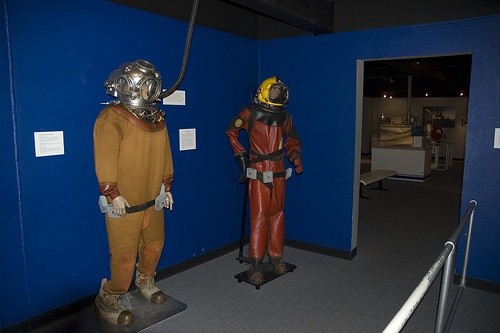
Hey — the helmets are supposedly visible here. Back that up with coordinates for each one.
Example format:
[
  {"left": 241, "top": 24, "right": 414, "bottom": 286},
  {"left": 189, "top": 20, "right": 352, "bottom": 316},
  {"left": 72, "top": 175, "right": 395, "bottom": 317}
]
[
  {"left": 103, "top": 59, "right": 162, "bottom": 120},
  {"left": 255, "top": 76, "right": 289, "bottom": 106}
]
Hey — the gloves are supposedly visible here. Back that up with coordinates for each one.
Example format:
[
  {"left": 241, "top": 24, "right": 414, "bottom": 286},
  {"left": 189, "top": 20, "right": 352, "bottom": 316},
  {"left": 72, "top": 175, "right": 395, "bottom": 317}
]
[
  {"left": 234, "top": 152, "right": 251, "bottom": 183},
  {"left": 290, "top": 152, "right": 303, "bottom": 174}
]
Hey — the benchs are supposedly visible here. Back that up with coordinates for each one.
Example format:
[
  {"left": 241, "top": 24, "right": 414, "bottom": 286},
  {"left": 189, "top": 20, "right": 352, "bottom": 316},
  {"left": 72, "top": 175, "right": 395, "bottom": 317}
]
[{"left": 359, "top": 169, "right": 397, "bottom": 200}]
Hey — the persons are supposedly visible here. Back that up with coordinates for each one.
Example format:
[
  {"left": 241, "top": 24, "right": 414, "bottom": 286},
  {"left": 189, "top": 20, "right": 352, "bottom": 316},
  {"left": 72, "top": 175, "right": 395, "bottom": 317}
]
[
  {"left": 93, "top": 60, "right": 173, "bottom": 326},
  {"left": 225, "top": 75, "right": 303, "bottom": 284}
]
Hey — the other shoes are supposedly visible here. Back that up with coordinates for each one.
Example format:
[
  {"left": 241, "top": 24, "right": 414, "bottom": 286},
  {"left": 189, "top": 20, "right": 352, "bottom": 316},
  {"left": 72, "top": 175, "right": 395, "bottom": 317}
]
[
  {"left": 248, "top": 258, "right": 264, "bottom": 285},
  {"left": 95, "top": 278, "right": 135, "bottom": 326},
  {"left": 269, "top": 256, "right": 286, "bottom": 273},
  {"left": 134, "top": 271, "right": 167, "bottom": 305}
]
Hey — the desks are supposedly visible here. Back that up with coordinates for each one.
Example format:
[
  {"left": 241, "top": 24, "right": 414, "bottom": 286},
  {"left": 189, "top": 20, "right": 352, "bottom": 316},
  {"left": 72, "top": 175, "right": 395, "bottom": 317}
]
[{"left": 371, "top": 147, "right": 432, "bottom": 182}]
